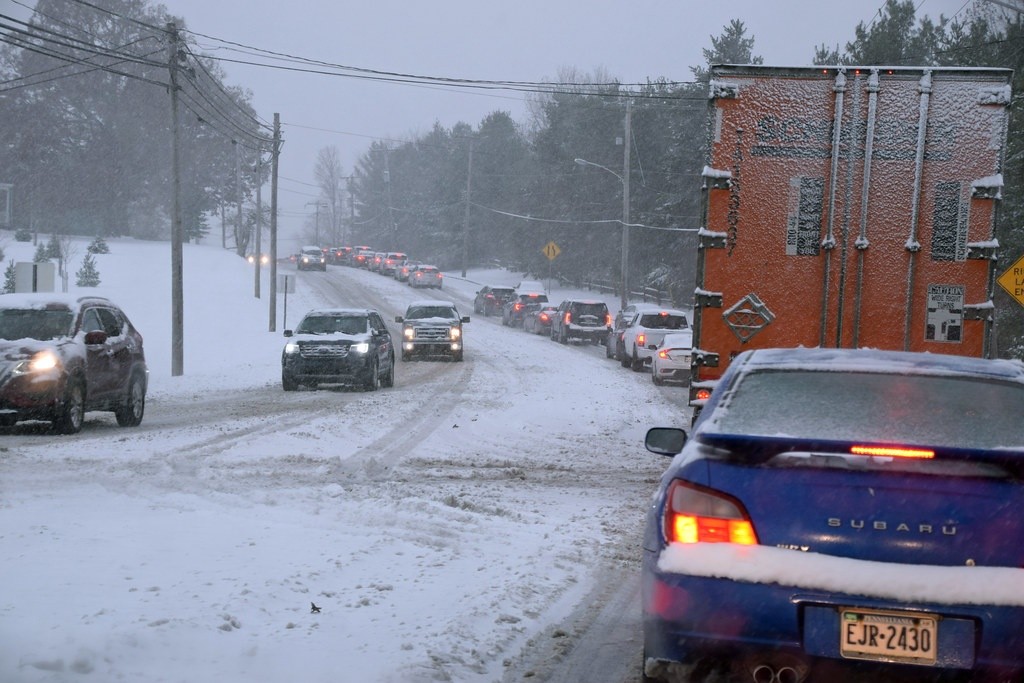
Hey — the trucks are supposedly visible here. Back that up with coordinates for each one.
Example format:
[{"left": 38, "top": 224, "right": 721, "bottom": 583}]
[{"left": 688, "top": 63, "right": 1015, "bottom": 431}]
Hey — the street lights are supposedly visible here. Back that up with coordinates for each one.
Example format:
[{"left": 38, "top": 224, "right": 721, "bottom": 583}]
[{"left": 574, "top": 97, "right": 632, "bottom": 310}]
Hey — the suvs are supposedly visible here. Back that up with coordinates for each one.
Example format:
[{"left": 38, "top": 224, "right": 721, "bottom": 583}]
[
  {"left": 502, "top": 291, "right": 549, "bottom": 328},
  {"left": 473, "top": 285, "right": 516, "bottom": 317},
  {"left": 281, "top": 309, "right": 395, "bottom": 392},
  {"left": 297, "top": 246, "right": 326, "bottom": 270},
  {"left": 550, "top": 300, "right": 612, "bottom": 346},
  {"left": 1, "top": 293, "right": 148, "bottom": 435},
  {"left": 620, "top": 310, "right": 693, "bottom": 372},
  {"left": 396, "top": 299, "right": 472, "bottom": 363}
]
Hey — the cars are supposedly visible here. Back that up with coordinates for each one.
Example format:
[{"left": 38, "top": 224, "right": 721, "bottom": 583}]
[
  {"left": 605, "top": 316, "right": 635, "bottom": 361},
  {"left": 642, "top": 348, "right": 1024, "bottom": 683},
  {"left": 614, "top": 302, "right": 662, "bottom": 326},
  {"left": 524, "top": 303, "right": 560, "bottom": 335},
  {"left": 648, "top": 334, "right": 692, "bottom": 388},
  {"left": 322, "top": 246, "right": 443, "bottom": 289}
]
